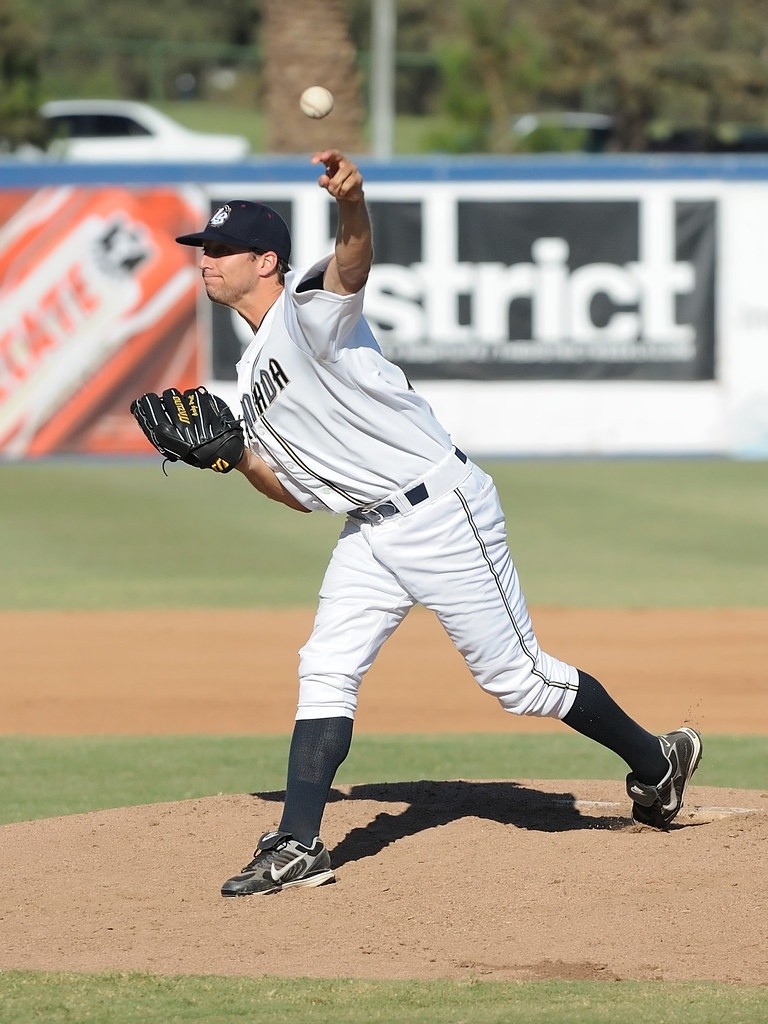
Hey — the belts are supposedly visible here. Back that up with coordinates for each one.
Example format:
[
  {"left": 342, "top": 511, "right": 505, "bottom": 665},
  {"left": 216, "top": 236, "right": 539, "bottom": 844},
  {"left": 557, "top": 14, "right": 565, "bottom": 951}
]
[{"left": 347, "top": 445, "right": 468, "bottom": 524}]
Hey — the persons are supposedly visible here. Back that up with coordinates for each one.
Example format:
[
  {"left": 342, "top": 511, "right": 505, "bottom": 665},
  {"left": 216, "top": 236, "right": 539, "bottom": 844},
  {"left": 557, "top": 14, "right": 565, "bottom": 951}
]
[{"left": 131, "top": 148, "right": 702, "bottom": 897}]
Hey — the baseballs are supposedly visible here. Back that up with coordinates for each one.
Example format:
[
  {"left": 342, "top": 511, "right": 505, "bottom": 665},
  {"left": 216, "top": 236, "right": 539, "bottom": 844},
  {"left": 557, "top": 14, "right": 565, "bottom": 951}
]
[{"left": 301, "top": 84, "right": 334, "bottom": 119}]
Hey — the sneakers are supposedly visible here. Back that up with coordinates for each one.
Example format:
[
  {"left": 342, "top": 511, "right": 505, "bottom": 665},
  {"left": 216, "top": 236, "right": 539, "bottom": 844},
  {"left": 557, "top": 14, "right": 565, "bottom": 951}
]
[
  {"left": 626, "top": 725, "right": 703, "bottom": 829},
  {"left": 222, "top": 830, "right": 335, "bottom": 900}
]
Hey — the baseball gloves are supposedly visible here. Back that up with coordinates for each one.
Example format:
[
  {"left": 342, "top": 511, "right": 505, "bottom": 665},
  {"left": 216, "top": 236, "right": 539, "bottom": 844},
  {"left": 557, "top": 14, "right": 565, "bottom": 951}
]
[{"left": 130, "top": 387, "right": 245, "bottom": 475}]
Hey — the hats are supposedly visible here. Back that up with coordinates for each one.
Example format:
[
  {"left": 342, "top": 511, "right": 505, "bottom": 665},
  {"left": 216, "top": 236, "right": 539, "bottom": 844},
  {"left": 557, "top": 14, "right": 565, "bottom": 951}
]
[{"left": 174, "top": 200, "right": 292, "bottom": 263}]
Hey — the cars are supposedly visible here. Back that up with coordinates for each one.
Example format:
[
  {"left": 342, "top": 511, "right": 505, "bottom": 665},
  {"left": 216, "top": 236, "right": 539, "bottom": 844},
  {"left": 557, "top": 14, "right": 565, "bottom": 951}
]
[
  {"left": 2, "top": 96, "right": 255, "bottom": 173},
  {"left": 460, "top": 112, "right": 767, "bottom": 156}
]
